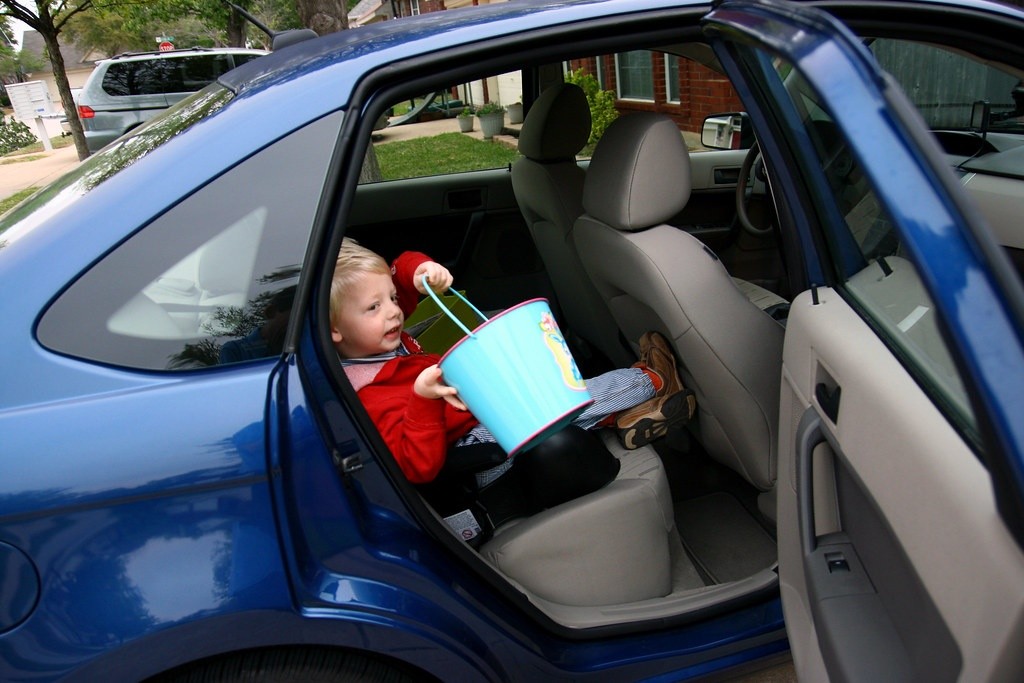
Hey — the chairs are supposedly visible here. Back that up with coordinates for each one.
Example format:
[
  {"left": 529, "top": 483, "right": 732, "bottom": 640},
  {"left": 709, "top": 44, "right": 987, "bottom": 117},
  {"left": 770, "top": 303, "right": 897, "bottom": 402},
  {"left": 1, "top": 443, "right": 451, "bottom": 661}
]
[{"left": 194, "top": 83, "right": 793, "bottom": 608}]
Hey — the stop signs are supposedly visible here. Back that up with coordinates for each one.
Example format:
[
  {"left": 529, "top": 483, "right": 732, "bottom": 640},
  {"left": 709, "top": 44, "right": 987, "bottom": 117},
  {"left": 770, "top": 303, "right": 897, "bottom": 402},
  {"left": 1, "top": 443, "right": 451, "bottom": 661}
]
[{"left": 159, "top": 41, "right": 174, "bottom": 51}]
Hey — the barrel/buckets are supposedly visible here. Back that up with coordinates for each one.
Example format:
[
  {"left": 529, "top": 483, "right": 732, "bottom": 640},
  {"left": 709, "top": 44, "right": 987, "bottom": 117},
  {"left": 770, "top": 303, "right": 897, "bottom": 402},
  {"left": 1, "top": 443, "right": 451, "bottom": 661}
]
[{"left": 421, "top": 274, "right": 596, "bottom": 459}]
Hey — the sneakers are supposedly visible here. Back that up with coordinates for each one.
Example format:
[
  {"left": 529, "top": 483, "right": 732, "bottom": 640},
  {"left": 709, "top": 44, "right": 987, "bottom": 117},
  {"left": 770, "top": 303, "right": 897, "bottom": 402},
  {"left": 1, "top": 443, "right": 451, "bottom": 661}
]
[
  {"left": 613, "top": 389, "right": 696, "bottom": 450},
  {"left": 629, "top": 330, "right": 684, "bottom": 398}
]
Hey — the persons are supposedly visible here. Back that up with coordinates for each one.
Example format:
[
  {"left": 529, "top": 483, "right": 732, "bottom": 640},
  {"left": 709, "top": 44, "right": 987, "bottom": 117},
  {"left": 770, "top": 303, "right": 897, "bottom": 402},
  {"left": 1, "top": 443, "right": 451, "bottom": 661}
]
[{"left": 331, "top": 240, "right": 697, "bottom": 487}]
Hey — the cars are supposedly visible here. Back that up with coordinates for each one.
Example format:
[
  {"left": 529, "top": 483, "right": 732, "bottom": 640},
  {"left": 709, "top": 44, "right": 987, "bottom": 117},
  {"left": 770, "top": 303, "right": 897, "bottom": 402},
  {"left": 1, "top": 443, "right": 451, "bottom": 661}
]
[{"left": 0, "top": 0, "right": 1024, "bottom": 683}]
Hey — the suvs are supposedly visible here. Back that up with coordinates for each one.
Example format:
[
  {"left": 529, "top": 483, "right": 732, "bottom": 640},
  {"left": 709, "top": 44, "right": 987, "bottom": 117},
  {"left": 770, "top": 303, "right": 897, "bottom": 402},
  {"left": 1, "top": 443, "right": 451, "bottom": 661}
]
[{"left": 77, "top": 44, "right": 274, "bottom": 155}]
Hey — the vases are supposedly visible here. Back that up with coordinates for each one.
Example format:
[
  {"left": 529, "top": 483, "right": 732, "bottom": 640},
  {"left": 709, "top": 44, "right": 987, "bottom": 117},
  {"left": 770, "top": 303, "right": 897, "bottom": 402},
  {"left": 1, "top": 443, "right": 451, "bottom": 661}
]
[{"left": 505, "top": 103, "right": 524, "bottom": 124}]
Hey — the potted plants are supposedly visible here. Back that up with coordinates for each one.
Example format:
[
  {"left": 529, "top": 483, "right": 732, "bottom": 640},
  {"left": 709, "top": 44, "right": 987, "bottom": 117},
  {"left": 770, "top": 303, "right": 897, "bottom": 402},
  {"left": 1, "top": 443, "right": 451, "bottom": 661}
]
[
  {"left": 455, "top": 106, "right": 475, "bottom": 133},
  {"left": 476, "top": 100, "right": 507, "bottom": 139}
]
